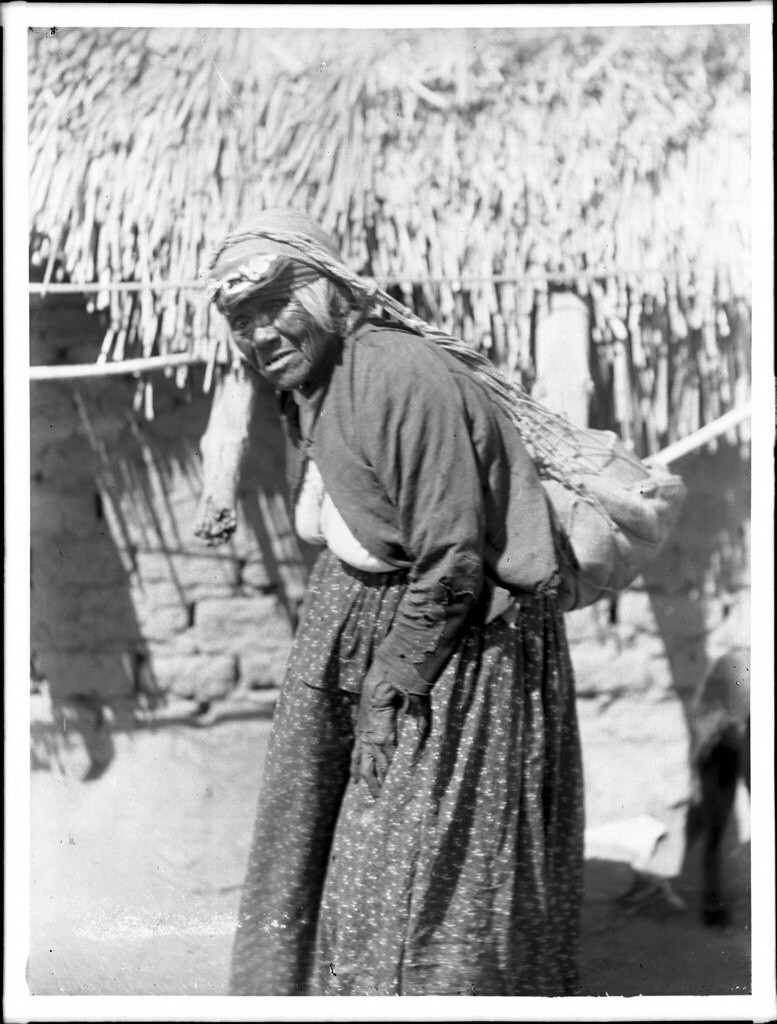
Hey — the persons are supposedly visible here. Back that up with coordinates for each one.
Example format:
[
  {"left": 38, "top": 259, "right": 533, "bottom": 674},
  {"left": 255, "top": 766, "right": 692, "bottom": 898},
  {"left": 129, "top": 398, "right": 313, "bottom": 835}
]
[{"left": 204, "top": 204, "right": 586, "bottom": 996}]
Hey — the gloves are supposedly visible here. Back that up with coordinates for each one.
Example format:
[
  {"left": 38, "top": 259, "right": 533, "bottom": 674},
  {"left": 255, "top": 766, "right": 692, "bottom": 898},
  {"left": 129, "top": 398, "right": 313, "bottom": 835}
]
[{"left": 345, "top": 657, "right": 399, "bottom": 797}]
